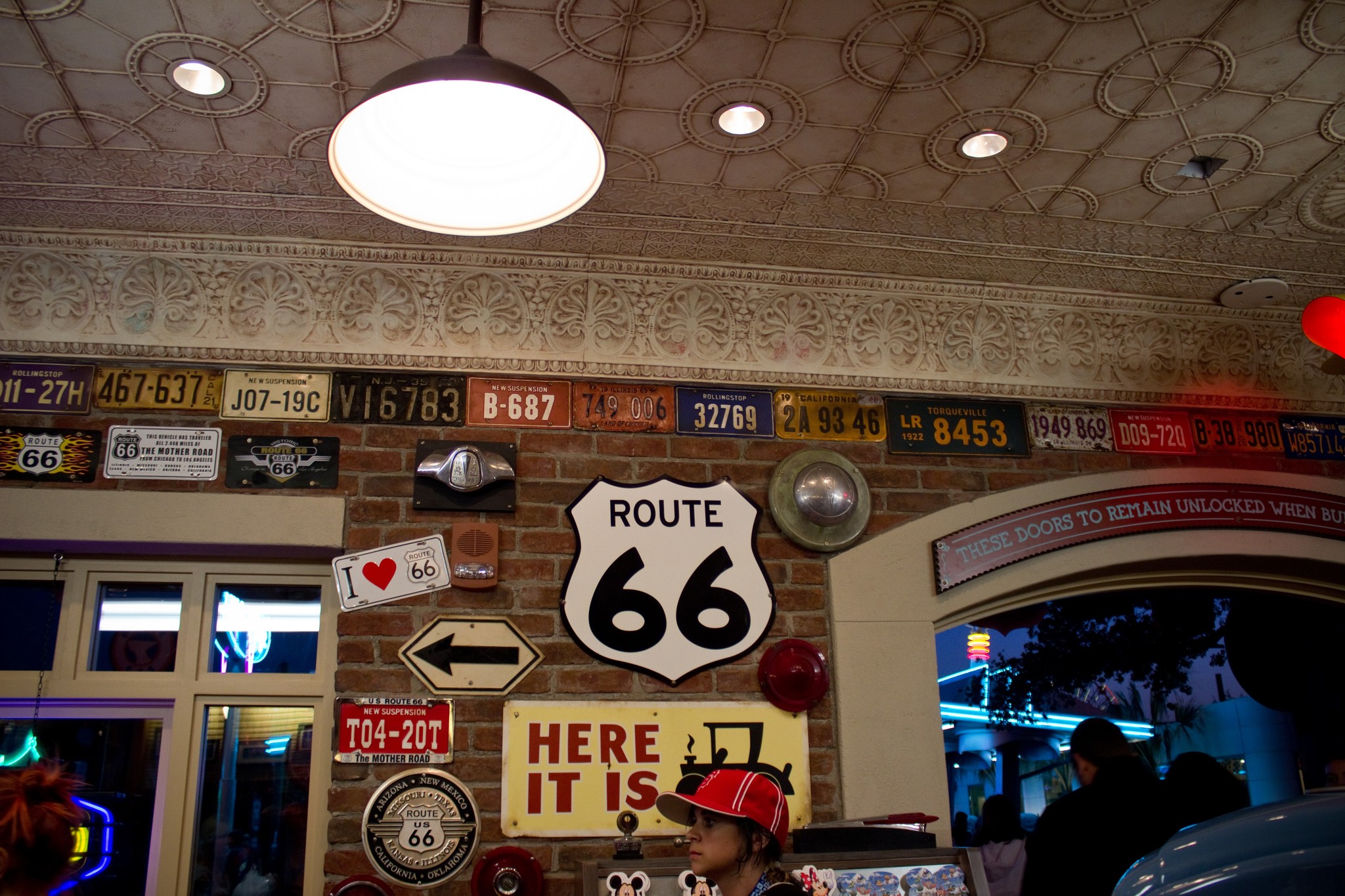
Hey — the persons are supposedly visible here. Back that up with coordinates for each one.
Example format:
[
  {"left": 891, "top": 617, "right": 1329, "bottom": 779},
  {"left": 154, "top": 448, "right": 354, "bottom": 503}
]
[
  {"left": 655, "top": 770, "right": 809, "bottom": 895},
  {"left": 0, "top": 757, "right": 85, "bottom": 896},
  {"left": 954, "top": 713, "right": 1247, "bottom": 895}
]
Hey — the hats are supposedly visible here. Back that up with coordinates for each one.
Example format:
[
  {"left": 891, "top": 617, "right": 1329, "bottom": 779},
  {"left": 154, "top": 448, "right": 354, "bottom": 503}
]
[{"left": 657, "top": 769, "right": 787, "bottom": 850}]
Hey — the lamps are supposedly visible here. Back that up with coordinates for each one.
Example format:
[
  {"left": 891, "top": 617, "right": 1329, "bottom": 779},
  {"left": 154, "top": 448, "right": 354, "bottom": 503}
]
[{"left": 327, "top": 1, "right": 607, "bottom": 237}]
